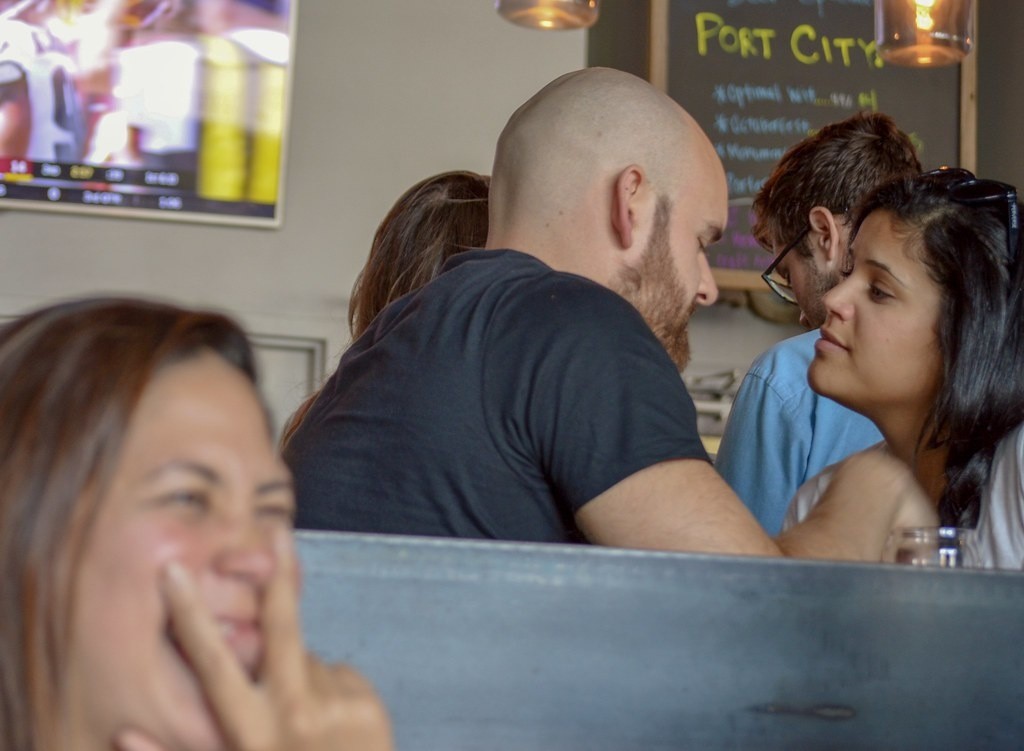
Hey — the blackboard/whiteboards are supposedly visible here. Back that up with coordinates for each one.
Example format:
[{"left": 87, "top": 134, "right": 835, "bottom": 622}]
[{"left": 650, "top": 0, "right": 978, "bottom": 290}]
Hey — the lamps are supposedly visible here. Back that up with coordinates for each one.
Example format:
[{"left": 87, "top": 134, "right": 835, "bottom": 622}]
[
  {"left": 492, "top": 0, "right": 599, "bottom": 30},
  {"left": 871, "top": 0, "right": 973, "bottom": 67}
]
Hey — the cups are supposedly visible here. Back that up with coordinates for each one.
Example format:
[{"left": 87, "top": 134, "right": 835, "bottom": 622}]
[{"left": 877, "top": 527, "right": 987, "bottom": 567}]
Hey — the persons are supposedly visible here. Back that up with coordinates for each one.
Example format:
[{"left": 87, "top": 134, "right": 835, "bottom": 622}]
[
  {"left": 0, "top": 296, "right": 393, "bottom": 751},
  {"left": 715, "top": 113, "right": 922, "bottom": 538},
  {"left": 782, "top": 168, "right": 1024, "bottom": 569},
  {"left": 279, "top": 66, "right": 941, "bottom": 563},
  {"left": 280, "top": 169, "right": 489, "bottom": 444}
]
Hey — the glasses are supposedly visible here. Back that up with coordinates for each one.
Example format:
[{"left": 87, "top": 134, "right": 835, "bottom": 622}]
[
  {"left": 759, "top": 206, "right": 849, "bottom": 305},
  {"left": 924, "top": 165, "right": 1019, "bottom": 282}
]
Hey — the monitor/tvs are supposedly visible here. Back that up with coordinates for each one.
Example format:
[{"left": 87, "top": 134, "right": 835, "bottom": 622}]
[{"left": 0, "top": 0, "right": 302, "bottom": 228}]
